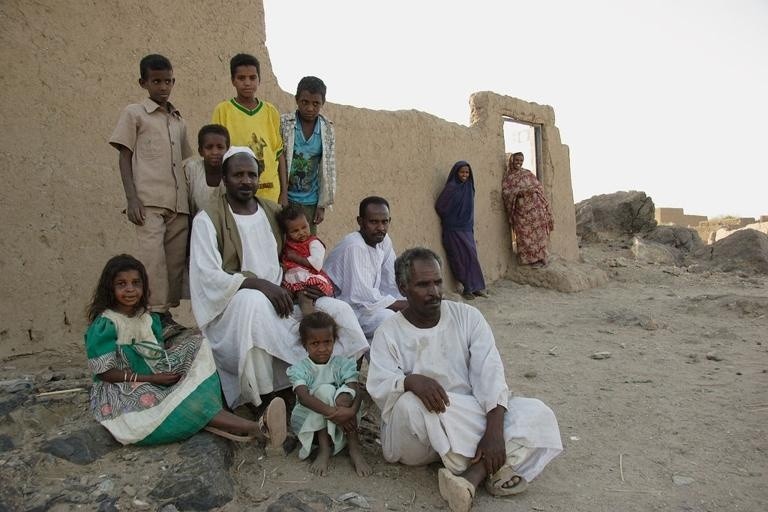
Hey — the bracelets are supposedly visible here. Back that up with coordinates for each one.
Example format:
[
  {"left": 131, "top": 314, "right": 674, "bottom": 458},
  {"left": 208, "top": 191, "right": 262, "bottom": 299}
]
[
  {"left": 134, "top": 372, "right": 138, "bottom": 383},
  {"left": 130, "top": 372, "right": 135, "bottom": 382},
  {"left": 123, "top": 370, "right": 128, "bottom": 383}
]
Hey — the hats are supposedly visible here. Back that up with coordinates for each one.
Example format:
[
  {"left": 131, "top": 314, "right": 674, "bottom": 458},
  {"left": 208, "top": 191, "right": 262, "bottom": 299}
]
[{"left": 222, "top": 145, "right": 257, "bottom": 164}]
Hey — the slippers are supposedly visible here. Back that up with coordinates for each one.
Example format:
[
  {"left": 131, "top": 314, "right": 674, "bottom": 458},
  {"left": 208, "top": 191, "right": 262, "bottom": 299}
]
[
  {"left": 485, "top": 465, "right": 528, "bottom": 496},
  {"left": 259, "top": 397, "right": 287, "bottom": 449},
  {"left": 438, "top": 468, "right": 475, "bottom": 511},
  {"left": 204, "top": 426, "right": 254, "bottom": 443}
]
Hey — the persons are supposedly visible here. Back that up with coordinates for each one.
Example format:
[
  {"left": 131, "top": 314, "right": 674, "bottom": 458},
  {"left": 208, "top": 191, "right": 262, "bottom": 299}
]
[
  {"left": 189, "top": 141, "right": 373, "bottom": 408},
  {"left": 323, "top": 195, "right": 412, "bottom": 351},
  {"left": 279, "top": 75, "right": 337, "bottom": 236},
  {"left": 272, "top": 203, "right": 337, "bottom": 316},
  {"left": 209, "top": 53, "right": 291, "bottom": 211},
  {"left": 82, "top": 252, "right": 287, "bottom": 449},
  {"left": 435, "top": 159, "right": 490, "bottom": 300},
  {"left": 181, "top": 124, "right": 231, "bottom": 219},
  {"left": 285, "top": 310, "right": 373, "bottom": 479},
  {"left": 501, "top": 152, "right": 554, "bottom": 269},
  {"left": 364, "top": 244, "right": 566, "bottom": 512},
  {"left": 106, "top": 53, "right": 199, "bottom": 339}
]
